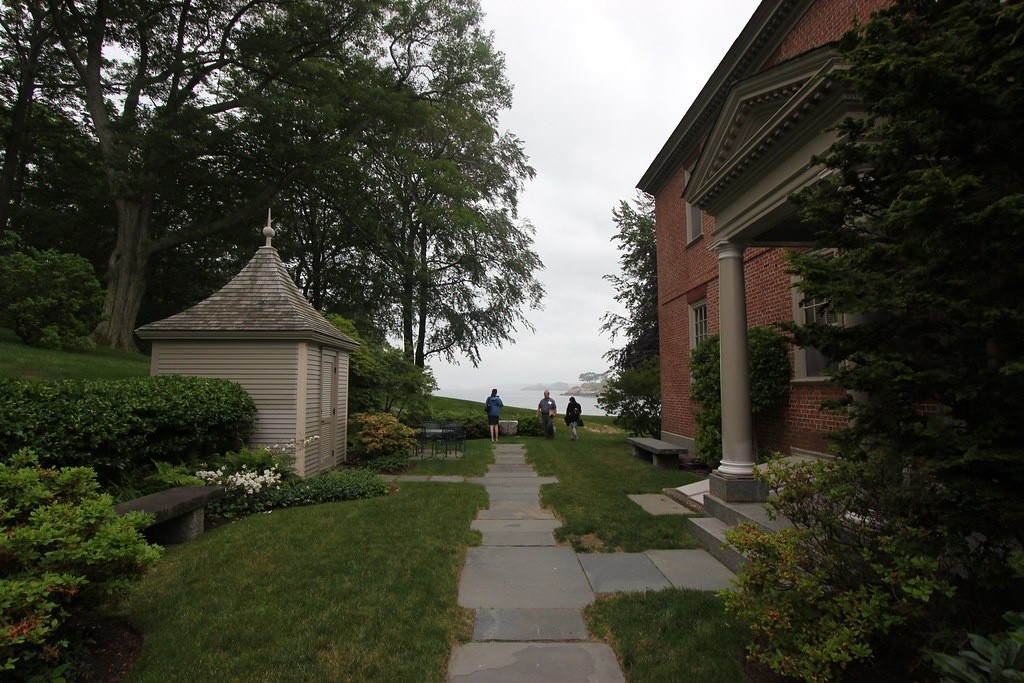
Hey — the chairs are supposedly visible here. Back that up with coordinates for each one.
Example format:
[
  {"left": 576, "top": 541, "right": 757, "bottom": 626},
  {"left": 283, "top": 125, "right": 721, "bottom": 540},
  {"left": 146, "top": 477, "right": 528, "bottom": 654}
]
[
  {"left": 407, "top": 425, "right": 427, "bottom": 461},
  {"left": 433, "top": 425, "right": 457, "bottom": 461}
]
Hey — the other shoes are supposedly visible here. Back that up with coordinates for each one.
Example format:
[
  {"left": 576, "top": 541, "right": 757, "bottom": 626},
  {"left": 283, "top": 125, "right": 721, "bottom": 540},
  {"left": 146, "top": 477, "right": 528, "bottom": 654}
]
[
  {"left": 548, "top": 435, "right": 554, "bottom": 439},
  {"left": 545, "top": 435, "right": 549, "bottom": 438}
]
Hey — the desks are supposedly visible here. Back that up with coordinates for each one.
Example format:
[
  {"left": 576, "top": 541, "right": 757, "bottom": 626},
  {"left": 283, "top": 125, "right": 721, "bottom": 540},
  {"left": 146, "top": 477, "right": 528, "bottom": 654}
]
[{"left": 417, "top": 429, "right": 455, "bottom": 461}]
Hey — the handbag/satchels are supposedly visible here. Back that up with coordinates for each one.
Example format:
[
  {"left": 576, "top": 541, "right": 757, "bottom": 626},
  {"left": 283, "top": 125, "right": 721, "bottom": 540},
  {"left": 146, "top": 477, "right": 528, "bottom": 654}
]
[
  {"left": 484, "top": 404, "right": 492, "bottom": 412},
  {"left": 577, "top": 419, "right": 584, "bottom": 427}
]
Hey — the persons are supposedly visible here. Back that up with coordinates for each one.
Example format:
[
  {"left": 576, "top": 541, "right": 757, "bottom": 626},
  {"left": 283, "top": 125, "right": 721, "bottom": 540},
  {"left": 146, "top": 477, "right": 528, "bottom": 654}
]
[
  {"left": 486, "top": 389, "right": 503, "bottom": 442},
  {"left": 536, "top": 390, "right": 557, "bottom": 439},
  {"left": 566, "top": 397, "right": 581, "bottom": 440}
]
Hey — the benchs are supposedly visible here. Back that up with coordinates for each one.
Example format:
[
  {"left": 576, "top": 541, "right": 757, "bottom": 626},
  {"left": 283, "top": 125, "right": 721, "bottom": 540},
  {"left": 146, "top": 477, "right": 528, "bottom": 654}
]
[
  {"left": 627, "top": 438, "right": 688, "bottom": 467},
  {"left": 113, "top": 486, "right": 226, "bottom": 542}
]
